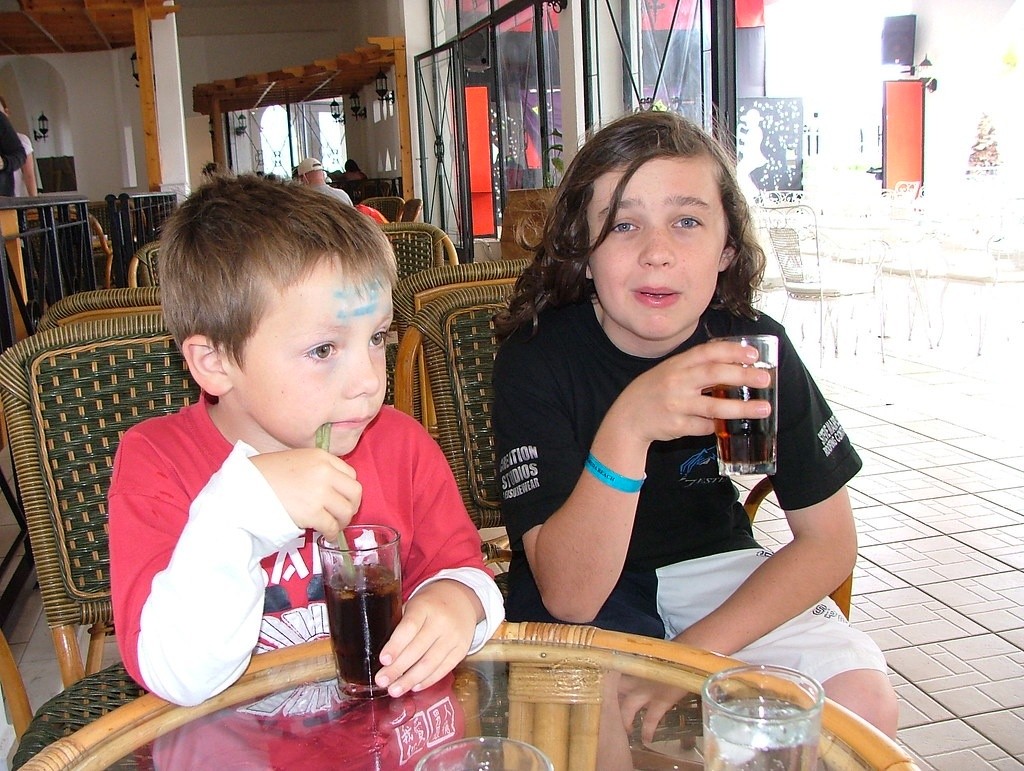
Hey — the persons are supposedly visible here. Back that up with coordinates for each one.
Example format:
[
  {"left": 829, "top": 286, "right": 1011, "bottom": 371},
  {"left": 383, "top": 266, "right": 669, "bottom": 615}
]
[
  {"left": 108, "top": 174, "right": 504, "bottom": 771},
  {"left": 491, "top": 109, "right": 898, "bottom": 771},
  {"left": 0, "top": 95, "right": 38, "bottom": 232},
  {"left": 257, "top": 159, "right": 368, "bottom": 208}
]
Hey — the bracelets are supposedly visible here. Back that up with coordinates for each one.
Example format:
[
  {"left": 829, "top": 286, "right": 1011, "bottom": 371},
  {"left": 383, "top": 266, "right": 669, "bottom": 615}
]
[{"left": 586, "top": 452, "right": 647, "bottom": 493}]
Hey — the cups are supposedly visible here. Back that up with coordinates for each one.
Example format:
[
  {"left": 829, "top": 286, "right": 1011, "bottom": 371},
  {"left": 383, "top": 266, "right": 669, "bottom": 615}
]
[
  {"left": 701, "top": 664, "right": 826, "bottom": 771},
  {"left": 707, "top": 333, "right": 779, "bottom": 475},
  {"left": 316, "top": 525, "right": 403, "bottom": 700}
]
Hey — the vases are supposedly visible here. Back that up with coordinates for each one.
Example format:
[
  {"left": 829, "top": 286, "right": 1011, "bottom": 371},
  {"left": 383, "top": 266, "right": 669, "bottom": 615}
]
[{"left": 544, "top": 172, "right": 554, "bottom": 189}]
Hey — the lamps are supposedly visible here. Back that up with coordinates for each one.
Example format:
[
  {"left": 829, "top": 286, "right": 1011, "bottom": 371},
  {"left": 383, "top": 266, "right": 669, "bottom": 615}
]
[
  {"left": 919, "top": 54, "right": 937, "bottom": 93},
  {"left": 375, "top": 66, "right": 395, "bottom": 106},
  {"left": 131, "top": 51, "right": 139, "bottom": 88},
  {"left": 34, "top": 111, "right": 49, "bottom": 142},
  {"left": 349, "top": 88, "right": 366, "bottom": 120},
  {"left": 330, "top": 100, "right": 346, "bottom": 125},
  {"left": 236, "top": 113, "right": 248, "bottom": 136}
]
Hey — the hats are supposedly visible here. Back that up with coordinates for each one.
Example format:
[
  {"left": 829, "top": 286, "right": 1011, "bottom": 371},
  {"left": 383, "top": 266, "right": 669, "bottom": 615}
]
[{"left": 298, "top": 157, "right": 323, "bottom": 176}]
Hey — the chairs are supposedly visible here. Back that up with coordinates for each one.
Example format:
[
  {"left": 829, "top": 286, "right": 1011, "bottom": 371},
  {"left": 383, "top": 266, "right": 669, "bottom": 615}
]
[{"left": 0, "top": 177, "right": 1024, "bottom": 771}]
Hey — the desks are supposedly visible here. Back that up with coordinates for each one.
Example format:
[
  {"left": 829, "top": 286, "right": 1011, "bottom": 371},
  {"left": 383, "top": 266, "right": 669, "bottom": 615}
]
[{"left": 16, "top": 622, "right": 919, "bottom": 771}]
